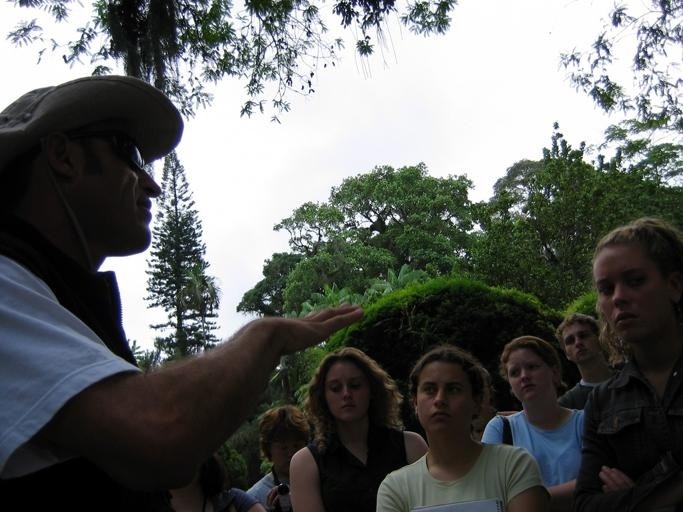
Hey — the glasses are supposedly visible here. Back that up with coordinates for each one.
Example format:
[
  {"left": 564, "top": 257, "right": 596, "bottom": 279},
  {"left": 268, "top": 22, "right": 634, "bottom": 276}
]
[{"left": 68, "top": 129, "right": 146, "bottom": 171}]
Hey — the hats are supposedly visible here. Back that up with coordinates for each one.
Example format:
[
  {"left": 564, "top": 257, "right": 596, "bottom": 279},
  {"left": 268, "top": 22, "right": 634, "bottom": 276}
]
[{"left": 0, "top": 75, "right": 183, "bottom": 172}]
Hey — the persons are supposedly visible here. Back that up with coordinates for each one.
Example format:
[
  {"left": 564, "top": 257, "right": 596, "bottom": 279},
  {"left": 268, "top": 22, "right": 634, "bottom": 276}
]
[
  {"left": 172, "top": 217, "right": 682, "bottom": 511},
  {"left": 0, "top": 76, "right": 364, "bottom": 512}
]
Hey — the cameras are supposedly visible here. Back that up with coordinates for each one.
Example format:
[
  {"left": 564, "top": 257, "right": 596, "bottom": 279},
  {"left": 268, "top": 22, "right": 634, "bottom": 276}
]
[{"left": 274, "top": 484, "right": 291, "bottom": 511}]
